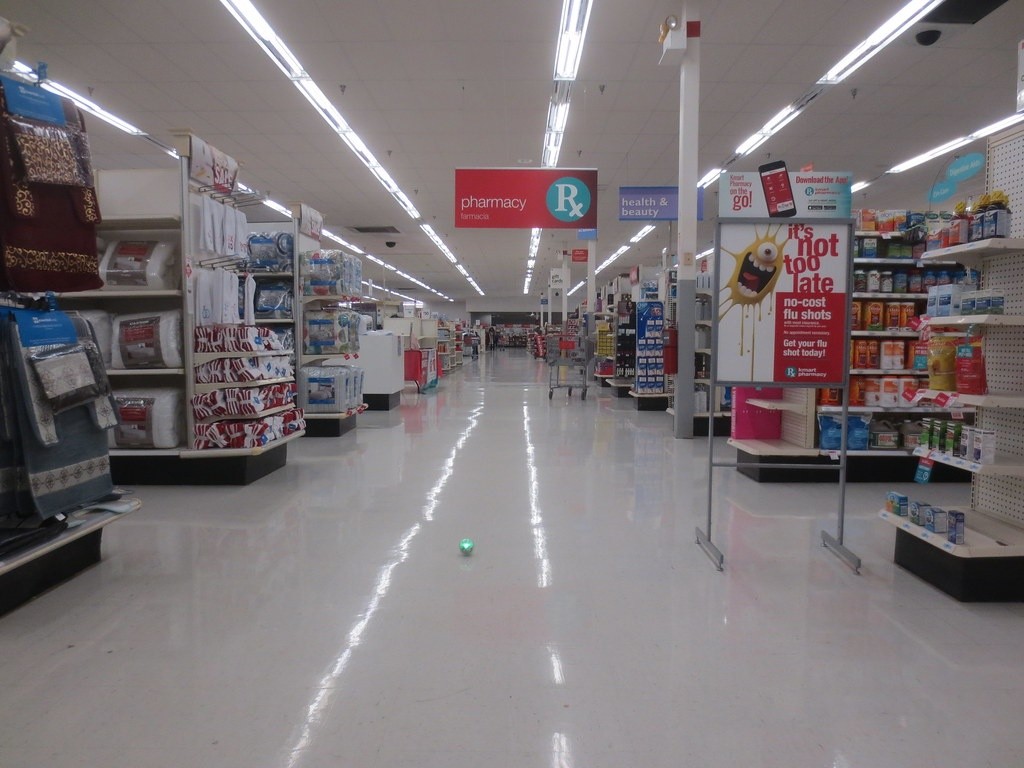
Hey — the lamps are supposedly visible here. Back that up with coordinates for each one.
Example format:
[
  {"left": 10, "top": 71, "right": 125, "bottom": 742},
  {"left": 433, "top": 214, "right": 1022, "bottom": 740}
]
[
  {"left": 289, "top": 77, "right": 352, "bottom": 133},
  {"left": 420, "top": 224, "right": 457, "bottom": 264},
  {"left": 10, "top": 61, "right": 293, "bottom": 218},
  {"left": 697, "top": 169, "right": 728, "bottom": 189},
  {"left": 735, "top": 105, "right": 801, "bottom": 156},
  {"left": 367, "top": 166, "right": 401, "bottom": 193},
  {"left": 595, "top": 246, "right": 630, "bottom": 276},
  {"left": 815, "top": 0, "right": 947, "bottom": 84},
  {"left": 391, "top": 190, "right": 421, "bottom": 220},
  {"left": 658, "top": 15, "right": 677, "bottom": 43},
  {"left": 529, "top": 227, "right": 542, "bottom": 258},
  {"left": 219, "top": 0, "right": 311, "bottom": 81},
  {"left": 336, "top": 126, "right": 383, "bottom": 168},
  {"left": 630, "top": 225, "right": 656, "bottom": 243}
]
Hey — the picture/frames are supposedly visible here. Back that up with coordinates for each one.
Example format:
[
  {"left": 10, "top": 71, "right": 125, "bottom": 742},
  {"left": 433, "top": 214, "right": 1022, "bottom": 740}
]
[{"left": 710, "top": 216, "right": 855, "bottom": 389}]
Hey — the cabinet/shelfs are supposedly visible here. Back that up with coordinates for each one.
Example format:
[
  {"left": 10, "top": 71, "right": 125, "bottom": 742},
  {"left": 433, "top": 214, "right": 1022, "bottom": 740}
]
[
  {"left": 237, "top": 221, "right": 297, "bottom": 410},
  {"left": 328, "top": 252, "right": 733, "bottom": 438},
  {"left": 0, "top": 167, "right": 186, "bottom": 455},
  {"left": 296, "top": 231, "right": 369, "bottom": 419},
  {"left": 877, "top": 120, "right": 1023, "bottom": 560},
  {"left": 180, "top": 155, "right": 305, "bottom": 458},
  {"left": 727, "top": 387, "right": 816, "bottom": 455},
  {"left": 813, "top": 232, "right": 967, "bottom": 456}
]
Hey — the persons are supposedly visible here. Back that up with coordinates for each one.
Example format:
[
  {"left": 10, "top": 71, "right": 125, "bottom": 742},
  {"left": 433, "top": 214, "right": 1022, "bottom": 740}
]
[{"left": 489, "top": 327, "right": 496, "bottom": 350}]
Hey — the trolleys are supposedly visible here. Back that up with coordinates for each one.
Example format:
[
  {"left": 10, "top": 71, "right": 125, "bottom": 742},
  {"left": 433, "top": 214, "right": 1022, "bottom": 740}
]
[
  {"left": 498, "top": 334, "right": 507, "bottom": 352},
  {"left": 546, "top": 332, "right": 590, "bottom": 401}
]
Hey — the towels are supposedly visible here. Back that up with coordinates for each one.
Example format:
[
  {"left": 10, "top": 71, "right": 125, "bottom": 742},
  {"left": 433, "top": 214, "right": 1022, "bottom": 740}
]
[
  {"left": 244, "top": 273, "right": 256, "bottom": 325},
  {"left": 196, "top": 267, "right": 240, "bottom": 325},
  {"left": 197, "top": 196, "right": 249, "bottom": 259}
]
